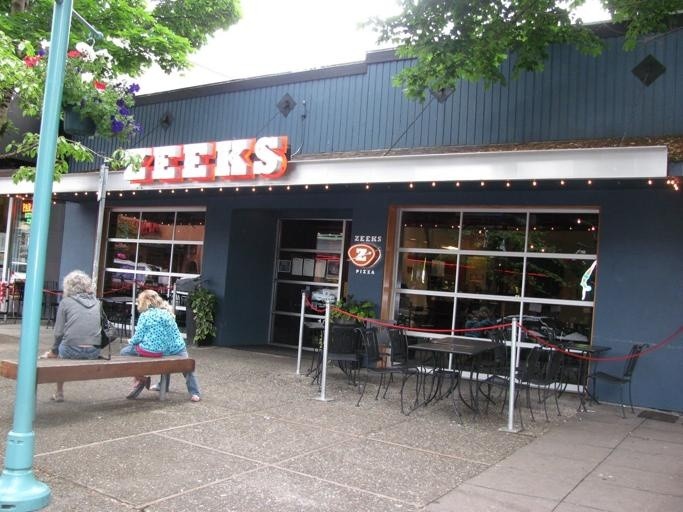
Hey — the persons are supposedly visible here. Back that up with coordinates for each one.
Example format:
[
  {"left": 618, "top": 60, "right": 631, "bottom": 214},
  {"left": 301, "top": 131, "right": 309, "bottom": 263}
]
[
  {"left": 117, "top": 287, "right": 179, "bottom": 402},
  {"left": 36, "top": 268, "right": 103, "bottom": 405},
  {"left": 465, "top": 307, "right": 496, "bottom": 339},
  {"left": 146, "top": 300, "right": 201, "bottom": 401}
]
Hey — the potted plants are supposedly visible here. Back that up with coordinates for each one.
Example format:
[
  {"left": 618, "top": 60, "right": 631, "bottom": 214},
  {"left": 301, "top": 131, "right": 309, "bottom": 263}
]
[{"left": 190, "top": 283, "right": 218, "bottom": 348}]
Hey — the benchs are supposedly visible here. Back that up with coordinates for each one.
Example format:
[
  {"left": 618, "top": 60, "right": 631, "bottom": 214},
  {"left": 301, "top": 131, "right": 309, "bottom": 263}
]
[{"left": 0, "top": 351, "right": 198, "bottom": 403}]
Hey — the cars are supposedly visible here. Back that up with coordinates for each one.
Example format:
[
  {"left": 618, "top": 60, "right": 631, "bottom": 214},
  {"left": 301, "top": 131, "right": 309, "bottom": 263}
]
[
  {"left": 110, "top": 259, "right": 201, "bottom": 325},
  {"left": 496, "top": 314, "right": 589, "bottom": 360},
  {"left": 11, "top": 261, "right": 28, "bottom": 282},
  {"left": 309, "top": 290, "right": 339, "bottom": 309},
  {"left": 395, "top": 294, "right": 423, "bottom": 364}
]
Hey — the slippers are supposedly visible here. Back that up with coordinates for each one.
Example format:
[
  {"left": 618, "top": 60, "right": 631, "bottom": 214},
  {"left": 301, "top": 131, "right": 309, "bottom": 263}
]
[
  {"left": 51, "top": 392, "right": 65, "bottom": 402},
  {"left": 38, "top": 350, "right": 63, "bottom": 359}
]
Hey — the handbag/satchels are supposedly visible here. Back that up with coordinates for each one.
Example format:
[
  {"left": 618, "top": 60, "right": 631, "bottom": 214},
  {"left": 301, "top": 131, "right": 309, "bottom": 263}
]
[{"left": 100, "top": 304, "right": 118, "bottom": 348}]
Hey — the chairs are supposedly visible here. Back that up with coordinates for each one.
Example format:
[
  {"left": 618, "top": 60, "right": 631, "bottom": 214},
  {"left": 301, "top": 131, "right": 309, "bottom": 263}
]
[{"left": 13, "top": 279, "right": 170, "bottom": 344}]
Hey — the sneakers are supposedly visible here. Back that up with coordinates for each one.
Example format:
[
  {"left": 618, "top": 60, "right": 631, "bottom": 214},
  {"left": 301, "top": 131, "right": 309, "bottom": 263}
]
[
  {"left": 145, "top": 376, "right": 152, "bottom": 392},
  {"left": 191, "top": 395, "right": 200, "bottom": 402},
  {"left": 150, "top": 384, "right": 168, "bottom": 391},
  {"left": 127, "top": 385, "right": 145, "bottom": 400}
]
